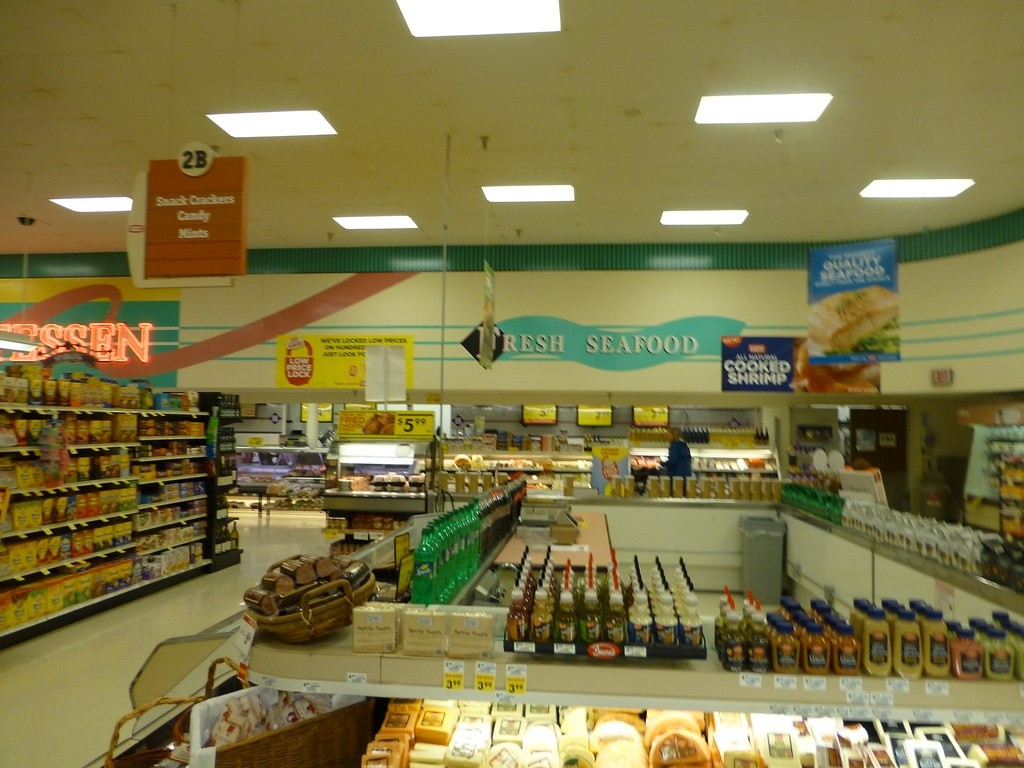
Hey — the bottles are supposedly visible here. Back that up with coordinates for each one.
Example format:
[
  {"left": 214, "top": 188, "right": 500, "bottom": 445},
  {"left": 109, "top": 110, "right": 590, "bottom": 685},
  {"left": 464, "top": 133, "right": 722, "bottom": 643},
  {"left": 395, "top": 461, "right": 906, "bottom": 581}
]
[
  {"left": 627, "top": 425, "right": 769, "bottom": 445},
  {"left": 219, "top": 427, "right": 236, "bottom": 453},
  {"left": 714, "top": 586, "right": 1024, "bottom": 680},
  {"left": 412, "top": 476, "right": 703, "bottom": 647},
  {"left": 217, "top": 458, "right": 237, "bottom": 488},
  {"left": 220, "top": 394, "right": 241, "bottom": 416},
  {"left": 584, "top": 433, "right": 602, "bottom": 451},
  {"left": 215, "top": 522, "right": 239, "bottom": 555},
  {"left": 217, "top": 496, "right": 228, "bottom": 521},
  {"left": 553, "top": 429, "right": 568, "bottom": 453}
]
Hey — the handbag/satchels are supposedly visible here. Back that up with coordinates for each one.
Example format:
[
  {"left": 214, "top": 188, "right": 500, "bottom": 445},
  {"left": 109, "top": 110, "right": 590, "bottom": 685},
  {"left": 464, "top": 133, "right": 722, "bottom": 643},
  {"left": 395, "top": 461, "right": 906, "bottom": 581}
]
[{"left": 631, "top": 466, "right": 659, "bottom": 482}]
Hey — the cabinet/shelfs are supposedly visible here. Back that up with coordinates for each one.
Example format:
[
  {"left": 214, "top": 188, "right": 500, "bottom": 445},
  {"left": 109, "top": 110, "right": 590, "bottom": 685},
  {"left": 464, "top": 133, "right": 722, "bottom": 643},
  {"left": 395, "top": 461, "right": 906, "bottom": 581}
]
[{"left": 0, "top": 392, "right": 244, "bottom": 652}]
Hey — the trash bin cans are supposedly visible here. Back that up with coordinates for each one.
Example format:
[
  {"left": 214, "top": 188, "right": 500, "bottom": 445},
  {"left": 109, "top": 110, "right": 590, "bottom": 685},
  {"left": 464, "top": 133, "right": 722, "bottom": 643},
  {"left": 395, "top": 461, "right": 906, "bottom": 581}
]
[{"left": 737, "top": 514, "right": 787, "bottom": 606}]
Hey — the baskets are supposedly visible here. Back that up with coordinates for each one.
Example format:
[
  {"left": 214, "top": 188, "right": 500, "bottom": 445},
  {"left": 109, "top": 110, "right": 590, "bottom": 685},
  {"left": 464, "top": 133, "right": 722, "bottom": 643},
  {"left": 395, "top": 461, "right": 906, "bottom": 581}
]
[
  {"left": 105, "top": 697, "right": 203, "bottom": 768},
  {"left": 245, "top": 554, "right": 376, "bottom": 642},
  {"left": 172, "top": 657, "right": 250, "bottom": 745},
  {"left": 191, "top": 687, "right": 374, "bottom": 768}
]
